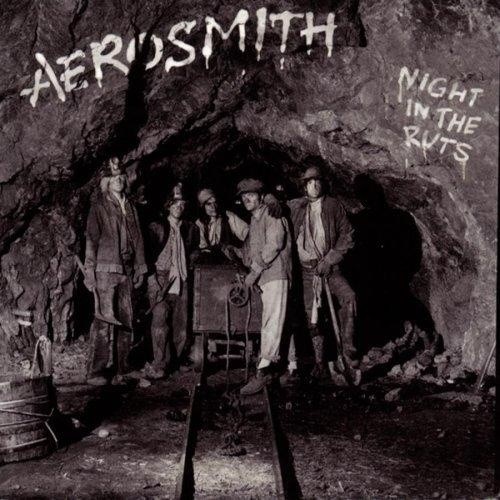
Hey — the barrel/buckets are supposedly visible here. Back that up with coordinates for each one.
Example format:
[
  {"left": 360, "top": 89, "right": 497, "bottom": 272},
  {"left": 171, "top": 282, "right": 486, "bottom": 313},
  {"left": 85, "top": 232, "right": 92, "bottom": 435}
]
[{"left": 1, "top": 372, "right": 61, "bottom": 463}]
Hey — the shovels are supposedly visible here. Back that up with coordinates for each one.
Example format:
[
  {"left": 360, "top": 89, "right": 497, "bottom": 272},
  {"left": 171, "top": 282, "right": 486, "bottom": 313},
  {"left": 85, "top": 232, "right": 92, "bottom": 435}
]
[
  {"left": 323, "top": 277, "right": 361, "bottom": 388},
  {"left": 73, "top": 255, "right": 123, "bottom": 326}
]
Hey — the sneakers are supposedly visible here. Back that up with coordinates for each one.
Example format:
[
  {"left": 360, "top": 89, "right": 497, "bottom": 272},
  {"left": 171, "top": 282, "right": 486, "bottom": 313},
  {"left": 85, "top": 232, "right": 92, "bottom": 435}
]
[
  {"left": 278, "top": 355, "right": 361, "bottom": 384},
  {"left": 85, "top": 362, "right": 166, "bottom": 390}
]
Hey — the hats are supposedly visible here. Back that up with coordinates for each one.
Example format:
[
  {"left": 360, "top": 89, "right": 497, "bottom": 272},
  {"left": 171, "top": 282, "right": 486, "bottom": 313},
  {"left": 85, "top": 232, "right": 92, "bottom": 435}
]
[
  {"left": 236, "top": 178, "right": 264, "bottom": 196},
  {"left": 197, "top": 189, "right": 215, "bottom": 206},
  {"left": 299, "top": 167, "right": 323, "bottom": 179}
]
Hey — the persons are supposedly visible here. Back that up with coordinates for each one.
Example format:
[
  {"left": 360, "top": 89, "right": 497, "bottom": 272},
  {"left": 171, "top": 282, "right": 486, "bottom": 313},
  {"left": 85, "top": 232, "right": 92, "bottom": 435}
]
[
  {"left": 142, "top": 180, "right": 192, "bottom": 379},
  {"left": 220, "top": 175, "right": 299, "bottom": 396},
  {"left": 186, "top": 188, "right": 250, "bottom": 266},
  {"left": 258, "top": 165, "right": 357, "bottom": 381},
  {"left": 82, "top": 155, "right": 147, "bottom": 386}
]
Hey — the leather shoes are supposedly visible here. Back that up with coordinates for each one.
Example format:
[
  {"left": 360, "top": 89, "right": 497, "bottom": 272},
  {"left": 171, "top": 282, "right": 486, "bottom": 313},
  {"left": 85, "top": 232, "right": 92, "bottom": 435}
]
[{"left": 238, "top": 375, "right": 271, "bottom": 394}]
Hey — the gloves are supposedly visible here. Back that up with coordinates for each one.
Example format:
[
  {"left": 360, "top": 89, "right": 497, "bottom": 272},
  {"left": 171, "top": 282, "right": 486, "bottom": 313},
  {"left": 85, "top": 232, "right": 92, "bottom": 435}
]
[{"left": 314, "top": 248, "right": 344, "bottom": 278}]
